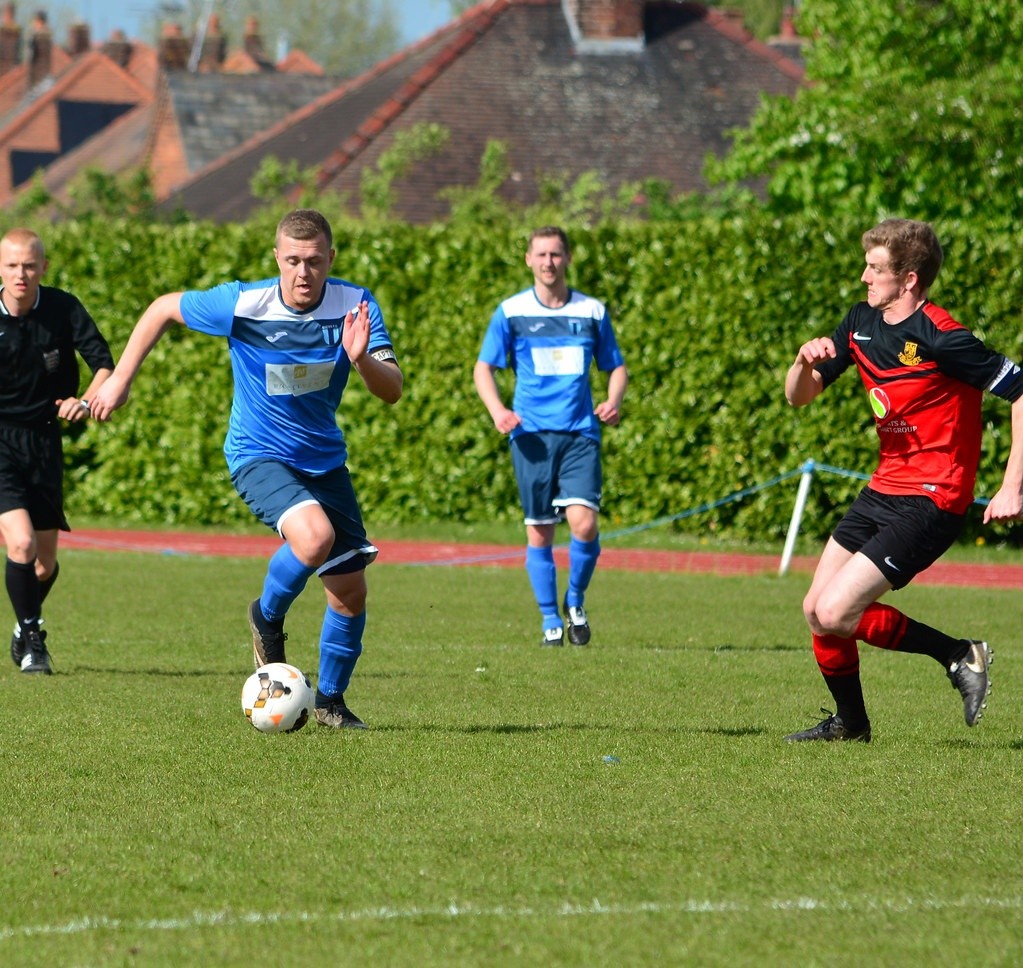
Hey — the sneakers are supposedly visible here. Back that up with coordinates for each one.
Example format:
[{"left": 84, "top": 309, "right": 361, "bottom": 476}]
[
  {"left": 783, "top": 708, "right": 871, "bottom": 744},
  {"left": 947, "top": 639, "right": 994, "bottom": 726},
  {"left": 22, "top": 633, "right": 52, "bottom": 676},
  {"left": 563, "top": 603, "right": 590, "bottom": 645},
  {"left": 248, "top": 598, "right": 288, "bottom": 670},
  {"left": 543, "top": 626, "right": 564, "bottom": 646},
  {"left": 11, "top": 622, "right": 25, "bottom": 666},
  {"left": 313, "top": 700, "right": 369, "bottom": 729}
]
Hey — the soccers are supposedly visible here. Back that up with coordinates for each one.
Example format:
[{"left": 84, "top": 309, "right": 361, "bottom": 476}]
[{"left": 241, "top": 662, "right": 315, "bottom": 735}]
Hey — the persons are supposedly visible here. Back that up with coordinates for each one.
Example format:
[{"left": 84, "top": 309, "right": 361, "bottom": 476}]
[
  {"left": 1, "top": 231, "right": 116, "bottom": 674},
  {"left": 86, "top": 210, "right": 404, "bottom": 732},
  {"left": 474, "top": 228, "right": 628, "bottom": 648},
  {"left": 780, "top": 217, "right": 1022, "bottom": 743}
]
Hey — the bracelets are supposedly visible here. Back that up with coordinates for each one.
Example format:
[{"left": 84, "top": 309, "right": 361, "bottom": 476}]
[{"left": 80, "top": 400, "right": 91, "bottom": 410}]
